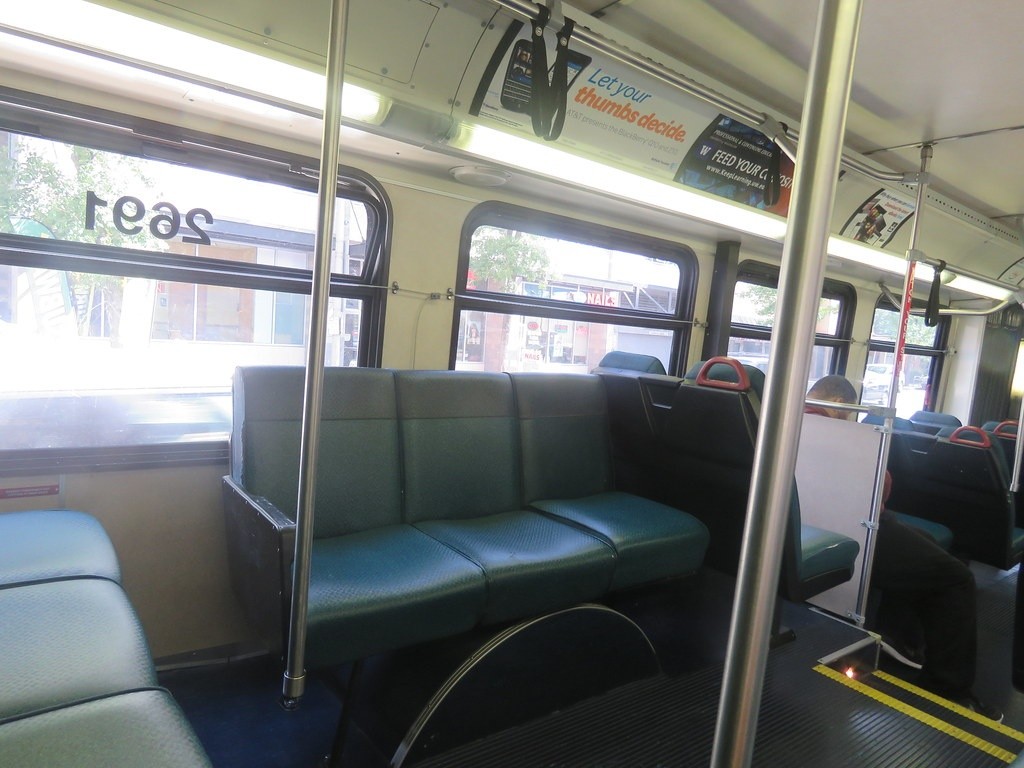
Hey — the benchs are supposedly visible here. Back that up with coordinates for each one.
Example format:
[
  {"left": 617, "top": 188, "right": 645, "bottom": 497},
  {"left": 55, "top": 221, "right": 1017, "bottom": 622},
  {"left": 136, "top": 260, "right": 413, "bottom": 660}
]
[{"left": 0, "top": 349, "right": 1024, "bottom": 768}]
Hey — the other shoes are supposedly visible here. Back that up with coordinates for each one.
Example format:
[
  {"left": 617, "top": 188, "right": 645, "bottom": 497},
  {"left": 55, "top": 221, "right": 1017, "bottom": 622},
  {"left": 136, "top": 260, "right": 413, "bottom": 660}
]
[
  {"left": 872, "top": 626, "right": 926, "bottom": 669},
  {"left": 963, "top": 700, "right": 1005, "bottom": 724}
]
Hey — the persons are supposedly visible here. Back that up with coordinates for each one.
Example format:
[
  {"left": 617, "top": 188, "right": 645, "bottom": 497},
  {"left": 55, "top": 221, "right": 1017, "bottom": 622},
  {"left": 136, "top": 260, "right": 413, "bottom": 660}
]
[
  {"left": 801, "top": 374, "right": 1005, "bottom": 722},
  {"left": 466, "top": 325, "right": 481, "bottom": 362}
]
